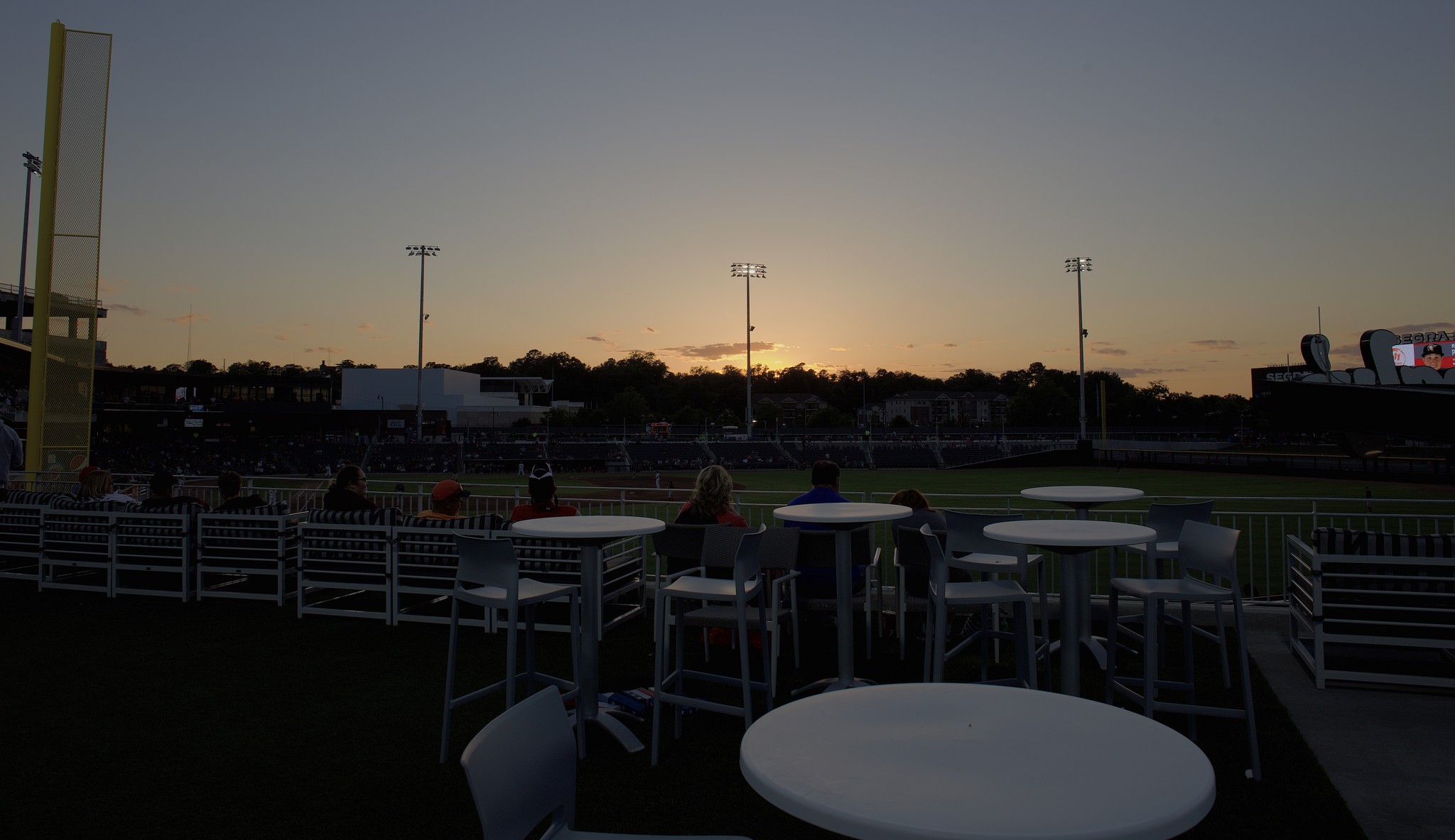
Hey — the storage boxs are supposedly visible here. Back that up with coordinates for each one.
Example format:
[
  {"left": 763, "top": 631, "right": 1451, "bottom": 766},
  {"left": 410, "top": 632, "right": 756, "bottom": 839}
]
[{"left": 608, "top": 685, "right": 706, "bottom": 715}]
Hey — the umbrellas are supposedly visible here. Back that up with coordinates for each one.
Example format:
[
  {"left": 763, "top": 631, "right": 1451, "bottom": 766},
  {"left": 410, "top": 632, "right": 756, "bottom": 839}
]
[{"left": 698, "top": 423, "right": 701, "bottom": 434}]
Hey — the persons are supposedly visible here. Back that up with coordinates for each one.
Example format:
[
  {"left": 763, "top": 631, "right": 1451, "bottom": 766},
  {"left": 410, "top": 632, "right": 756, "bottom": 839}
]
[
  {"left": 666, "top": 481, "right": 676, "bottom": 500},
  {"left": 73, "top": 466, "right": 141, "bottom": 504},
  {"left": 394, "top": 481, "right": 405, "bottom": 506},
  {"left": 140, "top": 471, "right": 213, "bottom": 510},
  {"left": 268, "top": 483, "right": 279, "bottom": 506},
  {"left": 0, "top": 419, "right": 23, "bottom": 488},
  {"left": 889, "top": 489, "right": 973, "bottom": 596},
  {"left": 417, "top": 480, "right": 471, "bottom": 520},
  {"left": 1364, "top": 485, "right": 1373, "bottom": 514},
  {"left": 367, "top": 429, "right": 1403, "bottom": 480},
  {"left": 652, "top": 470, "right": 663, "bottom": 489},
  {"left": 783, "top": 461, "right": 873, "bottom": 599},
  {"left": 322, "top": 465, "right": 378, "bottom": 510},
  {"left": 666, "top": 465, "right": 756, "bottom": 584},
  {"left": 0, "top": 379, "right": 372, "bottom": 484},
  {"left": 449, "top": 466, "right": 459, "bottom": 482},
  {"left": 213, "top": 471, "right": 267, "bottom": 510},
  {"left": 178, "top": 471, "right": 187, "bottom": 490},
  {"left": 508, "top": 462, "right": 582, "bottom": 522}
]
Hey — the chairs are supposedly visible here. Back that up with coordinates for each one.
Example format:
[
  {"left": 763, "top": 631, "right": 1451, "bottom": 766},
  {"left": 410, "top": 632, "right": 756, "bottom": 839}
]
[
  {"left": 0, "top": 488, "right": 1256, "bottom": 840},
  {"left": 1287, "top": 527, "right": 1455, "bottom": 693}
]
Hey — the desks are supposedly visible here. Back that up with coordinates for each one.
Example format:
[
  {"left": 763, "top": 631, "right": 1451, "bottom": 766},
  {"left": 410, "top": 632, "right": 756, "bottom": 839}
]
[
  {"left": 773, "top": 503, "right": 913, "bottom": 696},
  {"left": 1021, "top": 486, "right": 1144, "bottom": 669},
  {"left": 512, "top": 516, "right": 666, "bottom": 752},
  {"left": 983, "top": 520, "right": 1157, "bottom": 697},
  {"left": 740, "top": 683, "right": 1215, "bottom": 840}
]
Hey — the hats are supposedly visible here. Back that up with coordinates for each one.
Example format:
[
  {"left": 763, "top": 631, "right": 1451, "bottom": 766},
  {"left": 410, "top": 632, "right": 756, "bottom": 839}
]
[
  {"left": 149, "top": 470, "right": 178, "bottom": 489},
  {"left": 78, "top": 466, "right": 102, "bottom": 482},
  {"left": 1421, "top": 343, "right": 1444, "bottom": 358},
  {"left": 528, "top": 461, "right": 554, "bottom": 496},
  {"left": 430, "top": 479, "right": 471, "bottom": 501}
]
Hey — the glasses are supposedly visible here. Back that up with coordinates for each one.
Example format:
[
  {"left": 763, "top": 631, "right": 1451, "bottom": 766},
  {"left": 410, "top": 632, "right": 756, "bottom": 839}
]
[
  {"left": 354, "top": 477, "right": 367, "bottom": 483},
  {"left": 451, "top": 498, "right": 465, "bottom": 504},
  {"left": 109, "top": 479, "right": 114, "bottom": 485}
]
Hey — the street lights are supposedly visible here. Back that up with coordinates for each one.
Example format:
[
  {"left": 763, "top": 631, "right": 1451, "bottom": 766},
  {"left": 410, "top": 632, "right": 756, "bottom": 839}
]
[
  {"left": 956, "top": 407, "right": 970, "bottom": 436},
  {"left": 850, "top": 420, "right": 856, "bottom": 428},
  {"left": 730, "top": 263, "right": 768, "bottom": 440},
  {"left": 487, "top": 408, "right": 500, "bottom": 434},
  {"left": 719, "top": 404, "right": 732, "bottom": 432},
  {"left": 377, "top": 394, "right": 384, "bottom": 437},
  {"left": 876, "top": 407, "right": 890, "bottom": 437},
  {"left": 14, "top": 150, "right": 44, "bottom": 342},
  {"left": 797, "top": 408, "right": 811, "bottom": 437},
  {"left": 405, "top": 245, "right": 442, "bottom": 441},
  {"left": 640, "top": 408, "right": 654, "bottom": 425},
  {"left": 1064, "top": 256, "right": 1093, "bottom": 438},
  {"left": 563, "top": 407, "right": 577, "bottom": 438},
  {"left": 1127, "top": 408, "right": 1141, "bottom": 440},
  {"left": 1047, "top": 405, "right": 1062, "bottom": 440}
]
